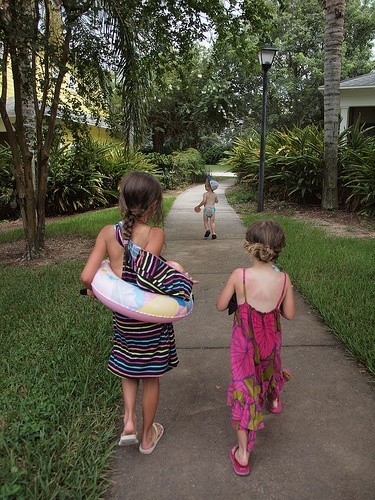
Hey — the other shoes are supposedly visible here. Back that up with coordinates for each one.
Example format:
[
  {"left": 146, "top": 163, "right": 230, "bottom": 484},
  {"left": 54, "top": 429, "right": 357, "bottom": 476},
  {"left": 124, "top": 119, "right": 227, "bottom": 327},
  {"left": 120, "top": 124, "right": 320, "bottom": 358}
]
[
  {"left": 212, "top": 234, "right": 216, "bottom": 239},
  {"left": 203, "top": 230, "right": 210, "bottom": 237}
]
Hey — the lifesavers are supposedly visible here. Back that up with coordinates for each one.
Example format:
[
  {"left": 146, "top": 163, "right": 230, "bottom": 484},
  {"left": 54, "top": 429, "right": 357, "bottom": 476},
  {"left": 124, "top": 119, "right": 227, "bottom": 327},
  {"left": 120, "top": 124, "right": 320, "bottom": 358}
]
[{"left": 87, "top": 258, "right": 195, "bottom": 325}]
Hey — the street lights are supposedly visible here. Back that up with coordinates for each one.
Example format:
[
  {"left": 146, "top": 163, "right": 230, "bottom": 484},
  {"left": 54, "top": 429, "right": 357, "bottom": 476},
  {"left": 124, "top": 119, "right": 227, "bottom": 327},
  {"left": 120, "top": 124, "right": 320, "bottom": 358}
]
[{"left": 254, "top": 35, "right": 280, "bottom": 214}]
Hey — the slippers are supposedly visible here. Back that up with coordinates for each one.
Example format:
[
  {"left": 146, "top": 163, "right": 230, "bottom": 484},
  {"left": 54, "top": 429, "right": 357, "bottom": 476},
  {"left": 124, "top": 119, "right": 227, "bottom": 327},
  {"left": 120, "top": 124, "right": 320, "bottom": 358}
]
[
  {"left": 118, "top": 431, "right": 139, "bottom": 447},
  {"left": 139, "top": 423, "right": 164, "bottom": 455},
  {"left": 229, "top": 445, "right": 250, "bottom": 476},
  {"left": 268, "top": 398, "right": 282, "bottom": 413}
]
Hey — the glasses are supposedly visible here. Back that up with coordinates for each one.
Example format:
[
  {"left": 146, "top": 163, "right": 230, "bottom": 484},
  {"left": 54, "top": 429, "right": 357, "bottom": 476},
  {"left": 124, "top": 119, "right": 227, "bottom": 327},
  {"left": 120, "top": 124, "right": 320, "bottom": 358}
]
[{"left": 205, "top": 185, "right": 210, "bottom": 187}]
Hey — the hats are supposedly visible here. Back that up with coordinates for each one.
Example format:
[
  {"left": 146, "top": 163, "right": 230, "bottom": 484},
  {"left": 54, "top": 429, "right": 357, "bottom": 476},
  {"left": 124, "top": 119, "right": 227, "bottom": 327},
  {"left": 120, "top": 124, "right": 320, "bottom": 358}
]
[{"left": 208, "top": 176, "right": 218, "bottom": 191}]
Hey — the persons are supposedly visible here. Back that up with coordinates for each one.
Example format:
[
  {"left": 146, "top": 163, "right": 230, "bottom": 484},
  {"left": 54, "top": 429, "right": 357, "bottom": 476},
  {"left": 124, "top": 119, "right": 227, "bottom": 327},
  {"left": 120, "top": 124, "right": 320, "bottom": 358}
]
[
  {"left": 80, "top": 171, "right": 200, "bottom": 454},
  {"left": 194, "top": 177, "right": 219, "bottom": 240},
  {"left": 216, "top": 219, "right": 294, "bottom": 476}
]
